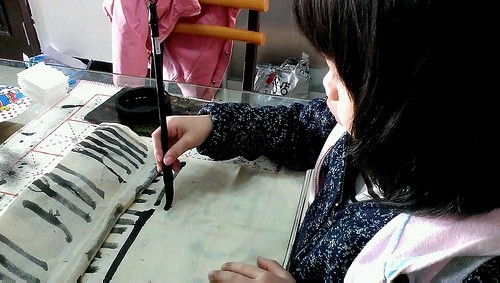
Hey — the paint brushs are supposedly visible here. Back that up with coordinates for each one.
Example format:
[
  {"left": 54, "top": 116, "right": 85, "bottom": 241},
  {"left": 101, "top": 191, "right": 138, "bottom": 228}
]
[{"left": 146, "top": 0, "right": 174, "bottom": 212}]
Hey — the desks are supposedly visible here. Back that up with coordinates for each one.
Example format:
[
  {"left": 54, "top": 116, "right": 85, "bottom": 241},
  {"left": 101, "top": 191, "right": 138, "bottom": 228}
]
[{"left": 0, "top": 59, "right": 312, "bottom": 283}]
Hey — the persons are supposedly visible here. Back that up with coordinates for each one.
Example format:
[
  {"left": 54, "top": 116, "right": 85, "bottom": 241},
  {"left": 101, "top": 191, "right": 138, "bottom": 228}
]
[{"left": 151, "top": 0, "right": 500, "bottom": 283}]
